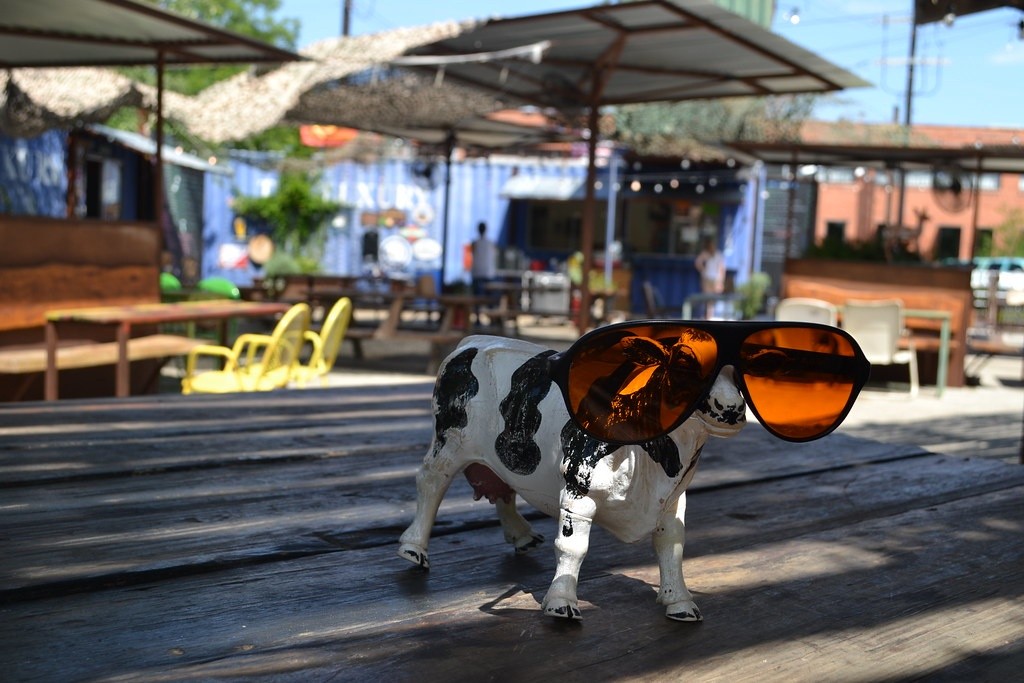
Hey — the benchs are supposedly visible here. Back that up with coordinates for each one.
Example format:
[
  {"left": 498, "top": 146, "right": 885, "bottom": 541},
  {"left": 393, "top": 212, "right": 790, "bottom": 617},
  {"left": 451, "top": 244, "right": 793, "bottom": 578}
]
[
  {"left": 0, "top": 211, "right": 215, "bottom": 401},
  {"left": 781, "top": 256, "right": 977, "bottom": 387},
  {"left": 344, "top": 327, "right": 464, "bottom": 346},
  {"left": 484, "top": 309, "right": 566, "bottom": 335}
]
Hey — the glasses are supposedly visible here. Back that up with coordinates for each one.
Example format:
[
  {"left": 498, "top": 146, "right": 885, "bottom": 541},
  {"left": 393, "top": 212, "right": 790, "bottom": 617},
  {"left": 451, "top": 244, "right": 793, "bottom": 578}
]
[{"left": 545, "top": 318, "right": 874, "bottom": 444}]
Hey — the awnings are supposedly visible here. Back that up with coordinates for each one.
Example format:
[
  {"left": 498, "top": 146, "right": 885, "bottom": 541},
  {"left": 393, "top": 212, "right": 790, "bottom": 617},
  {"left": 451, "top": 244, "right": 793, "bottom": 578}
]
[
  {"left": 90, "top": 124, "right": 233, "bottom": 174},
  {"left": 500, "top": 174, "right": 587, "bottom": 200}
]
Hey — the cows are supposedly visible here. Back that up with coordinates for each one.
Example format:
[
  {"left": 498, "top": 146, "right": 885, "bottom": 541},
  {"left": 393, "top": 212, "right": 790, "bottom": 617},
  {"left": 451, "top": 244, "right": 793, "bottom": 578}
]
[{"left": 393, "top": 328, "right": 797, "bottom": 626}]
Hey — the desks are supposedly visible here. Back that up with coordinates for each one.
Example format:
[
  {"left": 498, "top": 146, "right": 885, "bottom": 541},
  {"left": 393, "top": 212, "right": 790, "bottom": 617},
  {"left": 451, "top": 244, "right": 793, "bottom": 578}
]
[
  {"left": 487, "top": 279, "right": 572, "bottom": 334},
  {"left": 361, "top": 294, "right": 489, "bottom": 374},
  {"left": 43, "top": 299, "right": 293, "bottom": 398},
  {"left": 1, "top": 378, "right": 1024, "bottom": 683}
]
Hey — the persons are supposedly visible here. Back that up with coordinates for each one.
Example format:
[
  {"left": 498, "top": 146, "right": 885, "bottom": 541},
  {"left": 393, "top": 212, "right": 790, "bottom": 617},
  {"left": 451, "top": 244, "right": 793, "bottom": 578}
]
[
  {"left": 470, "top": 222, "right": 500, "bottom": 325},
  {"left": 695, "top": 235, "right": 726, "bottom": 320}
]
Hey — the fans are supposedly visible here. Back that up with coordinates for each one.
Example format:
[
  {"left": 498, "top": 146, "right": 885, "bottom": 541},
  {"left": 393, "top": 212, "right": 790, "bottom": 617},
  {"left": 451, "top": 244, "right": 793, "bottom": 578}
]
[{"left": 930, "top": 163, "right": 975, "bottom": 213}]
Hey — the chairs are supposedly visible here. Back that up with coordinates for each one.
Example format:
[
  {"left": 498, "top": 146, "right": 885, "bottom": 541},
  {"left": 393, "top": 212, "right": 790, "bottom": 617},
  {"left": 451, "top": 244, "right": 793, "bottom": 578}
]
[
  {"left": 775, "top": 297, "right": 839, "bottom": 328},
  {"left": 182, "top": 296, "right": 351, "bottom": 395},
  {"left": 840, "top": 298, "right": 919, "bottom": 395}
]
